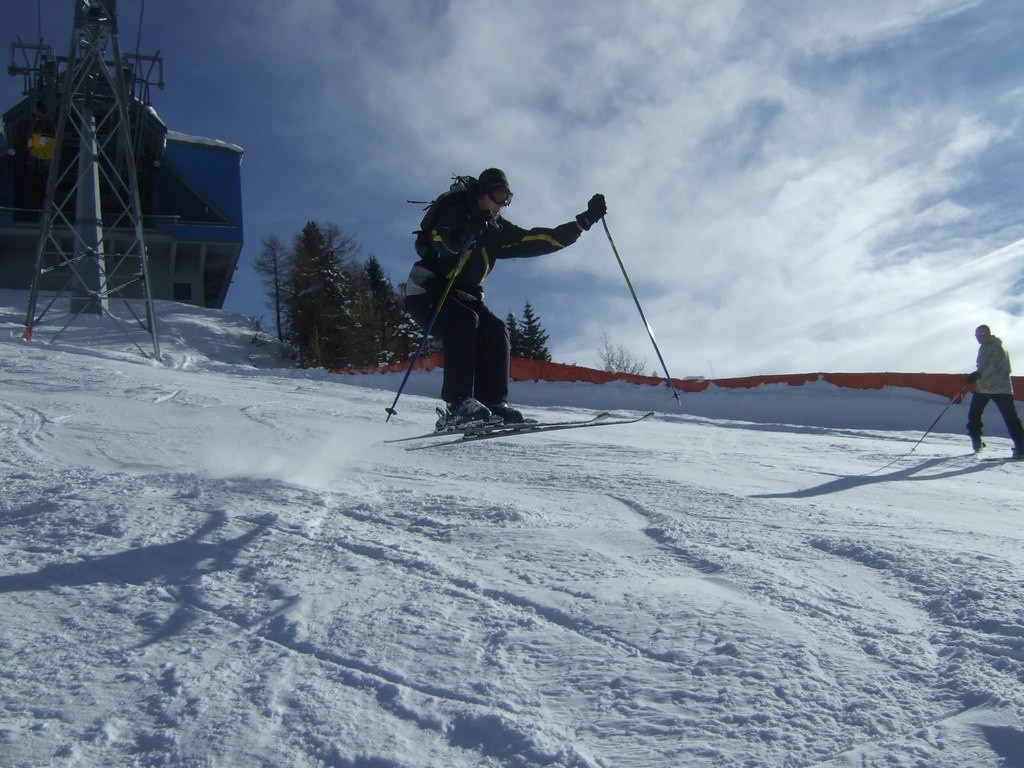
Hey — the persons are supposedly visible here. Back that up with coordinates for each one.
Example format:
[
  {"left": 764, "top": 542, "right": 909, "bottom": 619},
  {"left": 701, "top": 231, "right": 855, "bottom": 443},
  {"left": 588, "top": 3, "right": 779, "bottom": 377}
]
[
  {"left": 406, "top": 169, "right": 608, "bottom": 436},
  {"left": 967, "top": 325, "right": 1024, "bottom": 459}
]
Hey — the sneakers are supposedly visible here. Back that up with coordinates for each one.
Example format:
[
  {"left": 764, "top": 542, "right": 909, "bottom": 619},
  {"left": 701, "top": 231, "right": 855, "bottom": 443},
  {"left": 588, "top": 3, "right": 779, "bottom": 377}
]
[
  {"left": 446, "top": 400, "right": 492, "bottom": 422},
  {"left": 482, "top": 403, "right": 523, "bottom": 424}
]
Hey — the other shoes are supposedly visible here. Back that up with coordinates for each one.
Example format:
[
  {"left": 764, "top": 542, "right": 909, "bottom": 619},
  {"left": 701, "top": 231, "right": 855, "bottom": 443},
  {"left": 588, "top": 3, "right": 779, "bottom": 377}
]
[
  {"left": 1011, "top": 447, "right": 1024, "bottom": 458},
  {"left": 972, "top": 438, "right": 983, "bottom": 451}
]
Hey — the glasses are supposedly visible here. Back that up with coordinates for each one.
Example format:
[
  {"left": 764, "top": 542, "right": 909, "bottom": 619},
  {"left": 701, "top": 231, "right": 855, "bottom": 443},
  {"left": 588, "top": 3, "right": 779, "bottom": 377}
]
[{"left": 489, "top": 188, "right": 513, "bottom": 207}]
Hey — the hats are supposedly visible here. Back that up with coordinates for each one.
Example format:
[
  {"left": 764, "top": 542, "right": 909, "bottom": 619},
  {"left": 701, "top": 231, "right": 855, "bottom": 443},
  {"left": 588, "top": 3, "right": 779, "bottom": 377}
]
[{"left": 476, "top": 168, "right": 510, "bottom": 196}]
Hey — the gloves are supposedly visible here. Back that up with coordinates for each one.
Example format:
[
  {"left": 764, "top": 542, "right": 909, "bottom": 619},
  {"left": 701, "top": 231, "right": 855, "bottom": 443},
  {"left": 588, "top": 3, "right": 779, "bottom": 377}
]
[
  {"left": 967, "top": 372, "right": 981, "bottom": 383},
  {"left": 576, "top": 193, "right": 607, "bottom": 231},
  {"left": 468, "top": 209, "right": 494, "bottom": 234}
]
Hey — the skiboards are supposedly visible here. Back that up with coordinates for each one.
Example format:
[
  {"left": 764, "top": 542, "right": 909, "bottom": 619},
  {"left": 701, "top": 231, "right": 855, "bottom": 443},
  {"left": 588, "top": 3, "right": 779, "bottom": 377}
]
[{"left": 383, "top": 410, "right": 656, "bottom": 451}]
